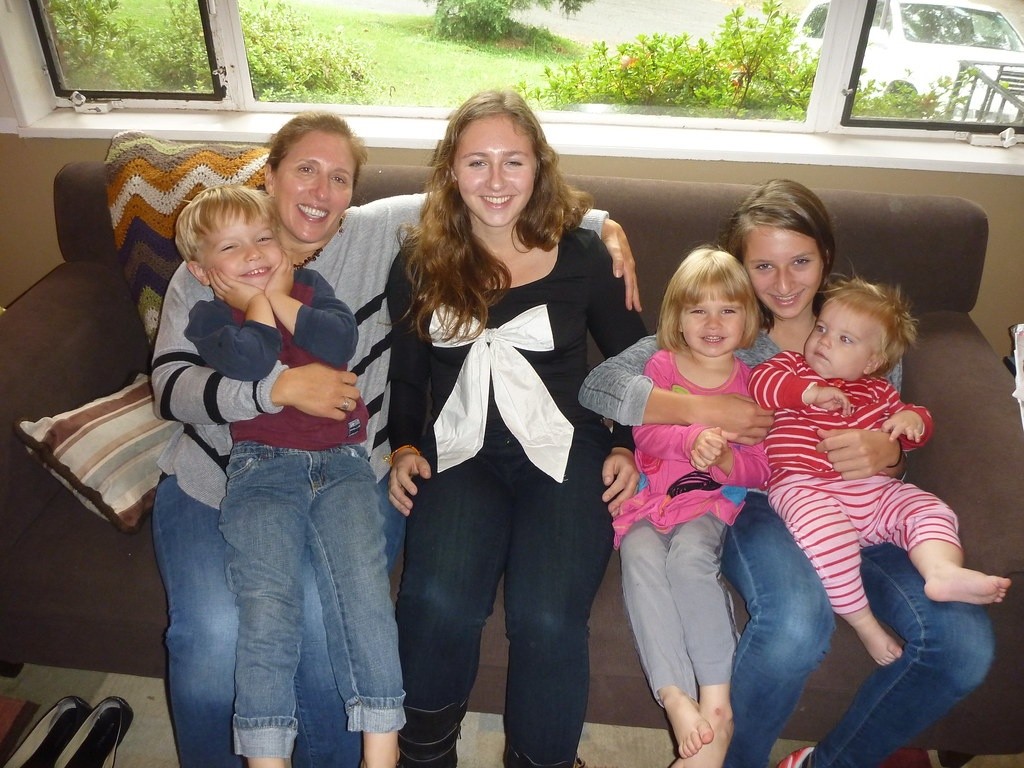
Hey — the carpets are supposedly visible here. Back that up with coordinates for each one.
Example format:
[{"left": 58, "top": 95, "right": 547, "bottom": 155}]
[{"left": 0, "top": 662, "right": 932, "bottom": 768}]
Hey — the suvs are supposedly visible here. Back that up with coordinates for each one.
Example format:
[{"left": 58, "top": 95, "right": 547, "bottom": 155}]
[{"left": 788, "top": 0, "right": 1024, "bottom": 123}]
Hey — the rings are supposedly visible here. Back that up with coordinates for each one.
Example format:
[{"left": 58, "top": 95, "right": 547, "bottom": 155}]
[{"left": 341, "top": 398, "right": 351, "bottom": 412}]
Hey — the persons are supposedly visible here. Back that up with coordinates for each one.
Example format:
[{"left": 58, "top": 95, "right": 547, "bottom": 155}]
[
  {"left": 148, "top": 108, "right": 647, "bottom": 766},
  {"left": 742, "top": 269, "right": 1012, "bottom": 668},
  {"left": 611, "top": 245, "right": 772, "bottom": 768},
  {"left": 173, "top": 183, "right": 409, "bottom": 767},
  {"left": 575, "top": 177, "right": 1012, "bottom": 767},
  {"left": 386, "top": 84, "right": 654, "bottom": 767}
]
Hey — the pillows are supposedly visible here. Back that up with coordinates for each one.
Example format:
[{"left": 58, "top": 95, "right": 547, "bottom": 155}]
[{"left": 13, "top": 373, "right": 182, "bottom": 535}]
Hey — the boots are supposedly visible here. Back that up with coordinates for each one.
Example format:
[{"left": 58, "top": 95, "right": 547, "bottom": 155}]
[
  {"left": 506, "top": 744, "right": 584, "bottom": 768},
  {"left": 397, "top": 696, "right": 469, "bottom": 768}
]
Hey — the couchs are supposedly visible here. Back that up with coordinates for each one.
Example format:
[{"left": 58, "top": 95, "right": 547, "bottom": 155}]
[{"left": 1, "top": 158, "right": 1023, "bottom": 768}]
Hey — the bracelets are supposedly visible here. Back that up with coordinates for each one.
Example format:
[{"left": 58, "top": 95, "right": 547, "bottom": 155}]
[{"left": 389, "top": 444, "right": 422, "bottom": 466}]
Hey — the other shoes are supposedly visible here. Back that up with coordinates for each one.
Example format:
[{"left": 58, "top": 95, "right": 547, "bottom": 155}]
[
  {"left": 0, "top": 696, "right": 92, "bottom": 768},
  {"left": 776, "top": 746, "right": 816, "bottom": 768},
  {"left": 51, "top": 696, "right": 134, "bottom": 768}
]
